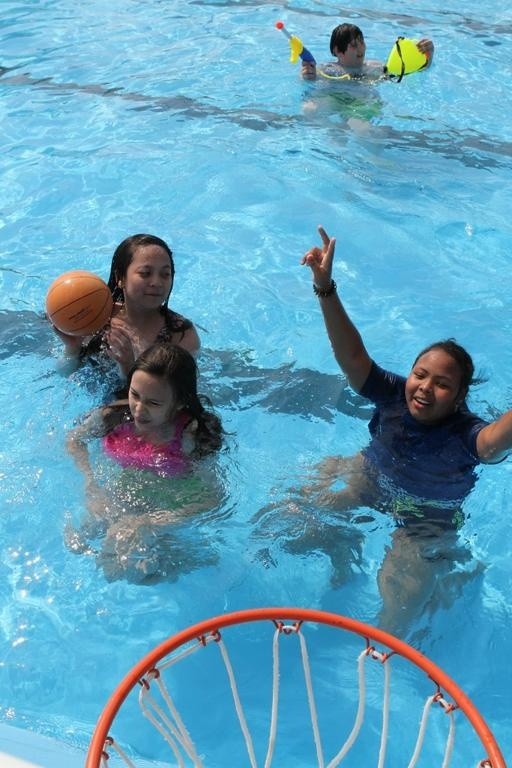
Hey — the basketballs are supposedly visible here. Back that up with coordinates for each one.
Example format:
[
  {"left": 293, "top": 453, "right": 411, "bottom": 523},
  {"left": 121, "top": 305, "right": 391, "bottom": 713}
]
[{"left": 46, "top": 271, "right": 113, "bottom": 337}]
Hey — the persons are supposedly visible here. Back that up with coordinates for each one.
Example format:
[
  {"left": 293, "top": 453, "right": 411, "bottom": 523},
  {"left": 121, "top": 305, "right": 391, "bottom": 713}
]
[
  {"left": 301, "top": 23, "right": 434, "bottom": 138},
  {"left": 44, "top": 235, "right": 200, "bottom": 382},
  {"left": 63, "top": 342, "right": 225, "bottom": 586},
  {"left": 283, "top": 226, "right": 512, "bottom": 638}
]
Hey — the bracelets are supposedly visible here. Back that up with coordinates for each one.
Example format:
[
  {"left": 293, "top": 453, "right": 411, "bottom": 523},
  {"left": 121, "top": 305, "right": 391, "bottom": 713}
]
[{"left": 313, "top": 280, "right": 335, "bottom": 297}]
